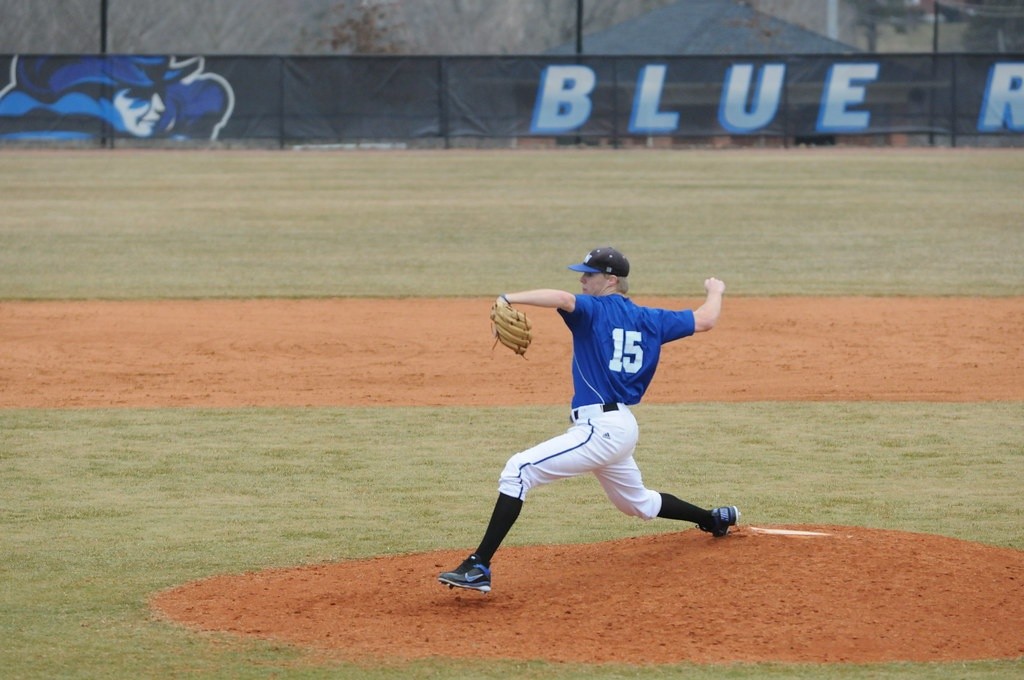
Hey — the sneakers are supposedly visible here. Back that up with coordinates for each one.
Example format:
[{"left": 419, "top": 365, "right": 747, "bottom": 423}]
[
  {"left": 438, "top": 554, "right": 492, "bottom": 592},
  {"left": 700, "top": 505, "right": 739, "bottom": 537}
]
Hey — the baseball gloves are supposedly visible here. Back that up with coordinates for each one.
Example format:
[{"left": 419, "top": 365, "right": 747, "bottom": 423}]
[{"left": 490, "top": 302, "right": 533, "bottom": 360}]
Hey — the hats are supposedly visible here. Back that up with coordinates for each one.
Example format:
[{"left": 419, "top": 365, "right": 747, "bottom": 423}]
[{"left": 567, "top": 247, "right": 629, "bottom": 277}]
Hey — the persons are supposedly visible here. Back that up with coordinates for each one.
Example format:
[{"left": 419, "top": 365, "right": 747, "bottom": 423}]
[{"left": 436, "top": 247, "right": 738, "bottom": 594}]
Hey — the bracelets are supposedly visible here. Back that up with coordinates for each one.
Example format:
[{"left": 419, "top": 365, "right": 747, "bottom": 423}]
[{"left": 498, "top": 293, "right": 511, "bottom": 306}]
